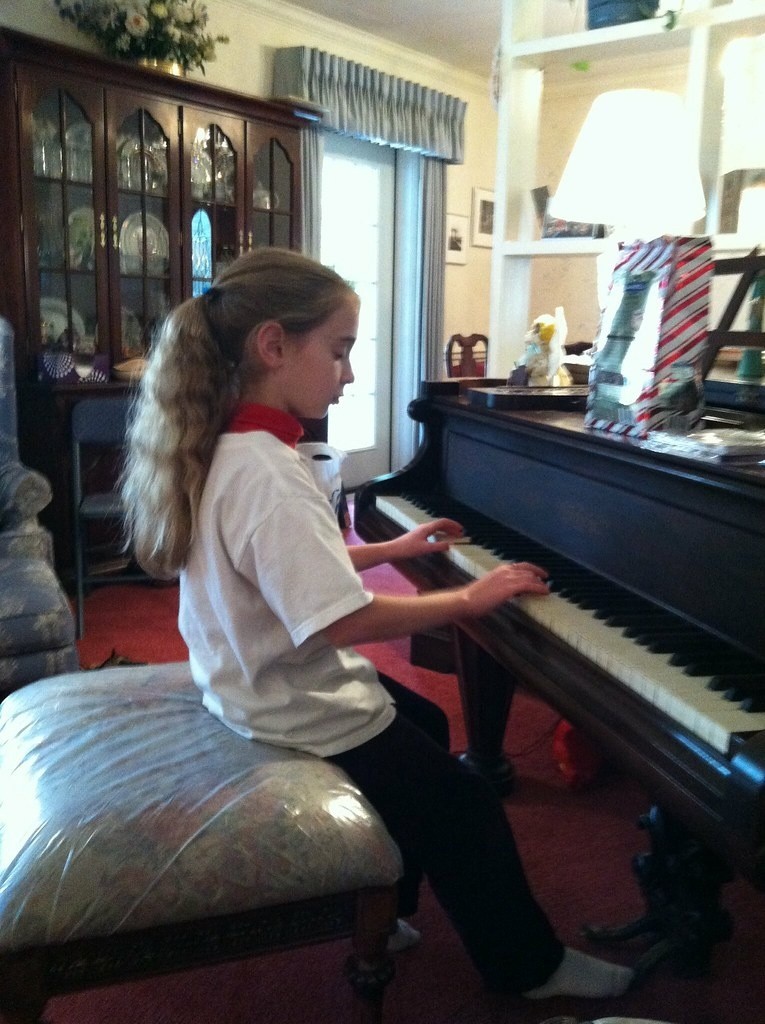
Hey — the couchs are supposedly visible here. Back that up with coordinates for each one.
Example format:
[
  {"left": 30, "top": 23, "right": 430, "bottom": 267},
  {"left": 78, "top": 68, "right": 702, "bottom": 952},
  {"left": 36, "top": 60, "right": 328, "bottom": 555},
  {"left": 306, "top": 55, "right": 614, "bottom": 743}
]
[{"left": 0, "top": 315, "right": 81, "bottom": 700}]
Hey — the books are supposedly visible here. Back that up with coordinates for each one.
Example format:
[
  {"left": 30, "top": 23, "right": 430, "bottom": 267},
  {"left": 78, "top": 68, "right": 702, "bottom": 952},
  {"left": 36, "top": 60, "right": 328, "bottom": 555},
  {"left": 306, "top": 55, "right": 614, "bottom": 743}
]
[{"left": 643, "top": 429, "right": 765, "bottom": 465}]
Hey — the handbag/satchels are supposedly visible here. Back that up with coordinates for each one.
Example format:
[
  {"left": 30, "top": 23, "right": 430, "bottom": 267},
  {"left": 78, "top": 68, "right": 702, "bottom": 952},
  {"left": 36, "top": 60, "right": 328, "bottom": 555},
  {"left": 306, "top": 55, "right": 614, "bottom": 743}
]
[{"left": 583, "top": 235, "right": 713, "bottom": 438}]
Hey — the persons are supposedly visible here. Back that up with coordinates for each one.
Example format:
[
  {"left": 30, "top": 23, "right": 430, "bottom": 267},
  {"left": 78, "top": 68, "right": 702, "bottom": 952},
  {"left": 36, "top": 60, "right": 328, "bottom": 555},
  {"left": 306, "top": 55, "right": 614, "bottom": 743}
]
[
  {"left": 449, "top": 228, "right": 462, "bottom": 249},
  {"left": 124, "top": 249, "right": 636, "bottom": 1000}
]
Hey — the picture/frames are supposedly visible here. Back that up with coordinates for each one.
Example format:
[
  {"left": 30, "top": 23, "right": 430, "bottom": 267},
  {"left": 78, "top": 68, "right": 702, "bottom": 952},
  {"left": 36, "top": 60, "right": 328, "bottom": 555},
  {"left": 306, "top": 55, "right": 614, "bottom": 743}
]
[
  {"left": 471, "top": 185, "right": 493, "bottom": 248},
  {"left": 446, "top": 213, "right": 469, "bottom": 266}
]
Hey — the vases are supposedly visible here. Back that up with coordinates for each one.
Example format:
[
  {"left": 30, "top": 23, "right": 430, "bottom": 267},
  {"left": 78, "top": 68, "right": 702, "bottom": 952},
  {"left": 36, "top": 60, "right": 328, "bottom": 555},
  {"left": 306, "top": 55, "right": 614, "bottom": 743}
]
[{"left": 136, "top": 58, "right": 184, "bottom": 78}]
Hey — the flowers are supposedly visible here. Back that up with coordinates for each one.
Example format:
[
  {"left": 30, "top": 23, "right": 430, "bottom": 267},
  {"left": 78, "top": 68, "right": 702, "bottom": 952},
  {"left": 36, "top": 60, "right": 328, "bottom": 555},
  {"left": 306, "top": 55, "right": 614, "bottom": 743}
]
[{"left": 54, "top": 0, "right": 230, "bottom": 77}]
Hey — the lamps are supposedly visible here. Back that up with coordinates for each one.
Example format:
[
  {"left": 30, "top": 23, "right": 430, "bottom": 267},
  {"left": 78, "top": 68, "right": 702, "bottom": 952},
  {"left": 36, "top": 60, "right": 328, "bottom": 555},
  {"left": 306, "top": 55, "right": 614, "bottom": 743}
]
[{"left": 549, "top": 88, "right": 708, "bottom": 244}]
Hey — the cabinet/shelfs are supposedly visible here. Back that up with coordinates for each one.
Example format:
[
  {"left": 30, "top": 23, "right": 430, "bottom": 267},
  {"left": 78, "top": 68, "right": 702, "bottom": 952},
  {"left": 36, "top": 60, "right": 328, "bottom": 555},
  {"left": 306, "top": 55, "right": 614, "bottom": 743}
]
[
  {"left": 0, "top": 25, "right": 310, "bottom": 595},
  {"left": 486, "top": 0, "right": 765, "bottom": 378}
]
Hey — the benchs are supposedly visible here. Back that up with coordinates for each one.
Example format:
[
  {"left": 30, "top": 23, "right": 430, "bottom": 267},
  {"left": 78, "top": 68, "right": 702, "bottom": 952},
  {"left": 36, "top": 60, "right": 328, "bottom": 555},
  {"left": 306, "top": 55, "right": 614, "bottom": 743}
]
[{"left": 0, "top": 660, "right": 407, "bottom": 1024}]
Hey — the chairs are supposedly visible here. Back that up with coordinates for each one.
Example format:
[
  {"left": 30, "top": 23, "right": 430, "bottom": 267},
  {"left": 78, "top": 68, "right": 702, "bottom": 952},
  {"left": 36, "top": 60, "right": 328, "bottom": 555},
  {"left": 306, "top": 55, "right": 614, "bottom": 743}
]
[{"left": 446, "top": 334, "right": 489, "bottom": 378}]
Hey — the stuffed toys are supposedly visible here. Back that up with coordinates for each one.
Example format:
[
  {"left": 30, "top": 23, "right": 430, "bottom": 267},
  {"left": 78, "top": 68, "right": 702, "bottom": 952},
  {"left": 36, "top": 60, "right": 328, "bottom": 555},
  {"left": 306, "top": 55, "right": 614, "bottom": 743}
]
[{"left": 513, "top": 307, "right": 573, "bottom": 386}]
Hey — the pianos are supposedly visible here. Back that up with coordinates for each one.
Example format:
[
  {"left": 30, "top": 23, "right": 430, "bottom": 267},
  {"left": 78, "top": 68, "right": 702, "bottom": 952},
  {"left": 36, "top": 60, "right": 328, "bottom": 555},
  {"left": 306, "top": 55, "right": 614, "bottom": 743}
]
[{"left": 349, "top": 360, "right": 765, "bottom": 987}]
